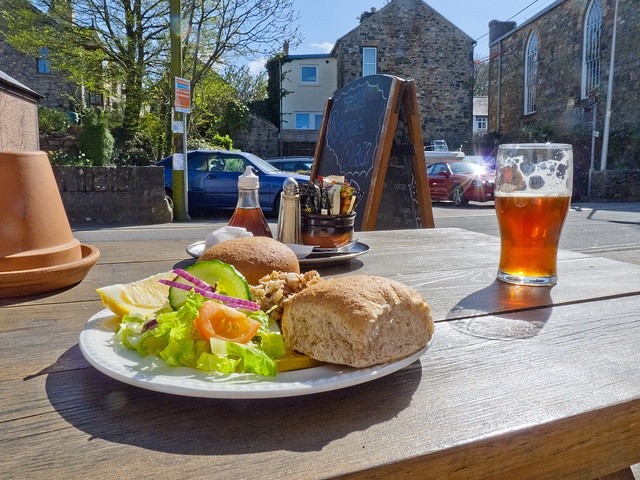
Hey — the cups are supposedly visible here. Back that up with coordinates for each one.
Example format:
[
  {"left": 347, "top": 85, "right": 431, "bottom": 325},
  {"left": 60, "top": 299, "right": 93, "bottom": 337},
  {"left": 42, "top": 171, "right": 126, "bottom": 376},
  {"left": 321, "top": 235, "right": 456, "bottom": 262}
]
[{"left": 493, "top": 142, "right": 574, "bottom": 285}]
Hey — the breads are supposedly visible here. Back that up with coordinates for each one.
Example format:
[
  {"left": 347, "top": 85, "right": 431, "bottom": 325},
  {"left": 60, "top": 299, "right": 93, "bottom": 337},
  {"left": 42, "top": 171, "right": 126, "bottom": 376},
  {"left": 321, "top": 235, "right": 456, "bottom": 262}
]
[
  {"left": 196, "top": 236, "right": 300, "bottom": 286},
  {"left": 279, "top": 273, "right": 435, "bottom": 370}
]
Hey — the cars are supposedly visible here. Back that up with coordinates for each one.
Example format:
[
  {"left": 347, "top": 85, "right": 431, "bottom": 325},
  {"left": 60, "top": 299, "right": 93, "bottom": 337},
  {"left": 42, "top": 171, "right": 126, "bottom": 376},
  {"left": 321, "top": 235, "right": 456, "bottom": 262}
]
[
  {"left": 427, "top": 160, "right": 498, "bottom": 207},
  {"left": 265, "top": 155, "right": 315, "bottom": 174},
  {"left": 154, "top": 151, "right": 310, "bottom": 211}
]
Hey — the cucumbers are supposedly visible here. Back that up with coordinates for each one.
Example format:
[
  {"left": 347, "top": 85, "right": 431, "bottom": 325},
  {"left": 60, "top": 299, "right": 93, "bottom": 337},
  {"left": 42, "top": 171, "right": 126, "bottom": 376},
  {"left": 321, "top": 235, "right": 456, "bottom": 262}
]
[{"left": 169, "top": 260, "right": 252, "bottom": 310}]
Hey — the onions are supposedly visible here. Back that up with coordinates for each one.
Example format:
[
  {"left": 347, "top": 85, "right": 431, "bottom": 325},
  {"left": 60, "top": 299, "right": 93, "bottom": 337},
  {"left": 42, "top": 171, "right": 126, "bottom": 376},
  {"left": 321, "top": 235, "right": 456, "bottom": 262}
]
[{"left": 155, "top": 266, "right": 261, "bottom": 313}]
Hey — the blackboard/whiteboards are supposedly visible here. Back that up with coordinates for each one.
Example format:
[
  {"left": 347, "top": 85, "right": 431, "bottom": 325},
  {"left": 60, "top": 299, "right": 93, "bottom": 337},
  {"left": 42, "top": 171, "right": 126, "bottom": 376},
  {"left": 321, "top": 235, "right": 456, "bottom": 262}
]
[
  {"left": 376, "top": 78, "right": 436, "bottom": 230},
  {"left": 308, "top": 73, "right": 407, "bottom": 232}
]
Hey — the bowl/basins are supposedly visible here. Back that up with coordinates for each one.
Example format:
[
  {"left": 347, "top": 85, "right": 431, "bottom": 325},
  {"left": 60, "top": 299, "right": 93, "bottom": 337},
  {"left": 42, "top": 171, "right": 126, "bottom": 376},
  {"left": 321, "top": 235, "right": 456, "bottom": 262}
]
[{"left": 300, "top": 214, "right": 357, "bottom": 245}]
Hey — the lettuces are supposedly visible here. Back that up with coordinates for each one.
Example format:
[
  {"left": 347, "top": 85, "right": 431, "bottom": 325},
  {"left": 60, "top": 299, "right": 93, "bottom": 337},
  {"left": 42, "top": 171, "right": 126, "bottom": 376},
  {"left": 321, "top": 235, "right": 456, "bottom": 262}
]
[{"left": 116, "top": 290, "right": 288, "bottom": 383}]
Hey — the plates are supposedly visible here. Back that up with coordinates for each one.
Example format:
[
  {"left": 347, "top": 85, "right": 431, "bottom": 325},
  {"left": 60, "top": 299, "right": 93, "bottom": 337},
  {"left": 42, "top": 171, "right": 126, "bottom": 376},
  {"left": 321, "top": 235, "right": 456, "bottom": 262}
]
[
  {"left": 78, "top": 296, "right": 435, "bottom": 402},
  {"left": 186, "top": 239, "right": 371, "bottom": 265}
]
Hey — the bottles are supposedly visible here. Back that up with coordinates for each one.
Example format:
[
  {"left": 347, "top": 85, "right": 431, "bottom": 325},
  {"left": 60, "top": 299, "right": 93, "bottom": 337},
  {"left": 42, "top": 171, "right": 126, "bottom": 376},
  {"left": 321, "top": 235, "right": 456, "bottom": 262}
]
[
  {"left": 226, "top": 165, "right": 274, "bottom": 238},
  {"left": 276, "top": 177, "right": 303, "bottom": 244}
]
